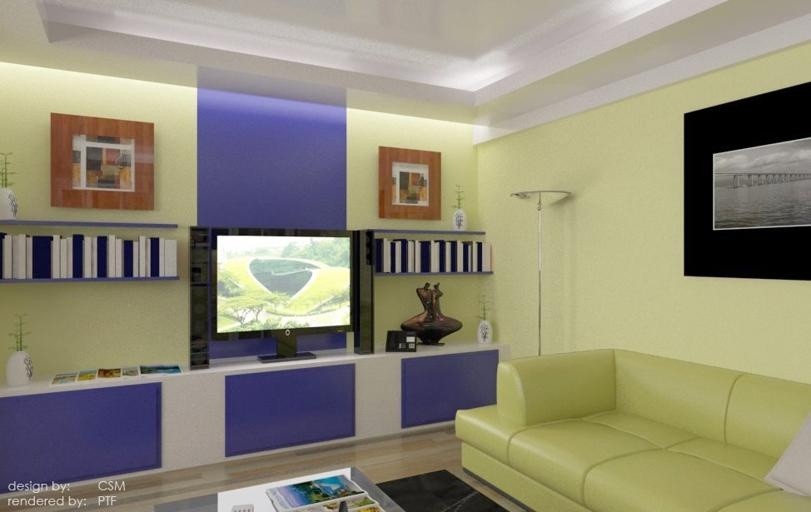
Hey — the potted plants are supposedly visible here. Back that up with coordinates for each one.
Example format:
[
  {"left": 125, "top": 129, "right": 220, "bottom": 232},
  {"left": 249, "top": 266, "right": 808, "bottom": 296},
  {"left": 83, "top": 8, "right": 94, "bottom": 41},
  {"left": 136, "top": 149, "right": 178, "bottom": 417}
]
[
  {"left": 4, "top": 312, "right": 35, "bottom": 386},
  {"left": 1, "top": 151, "right": 18, "bottom": 220},
  {"left": 476, "top": 291, "right": 496, "bottom": 345},
  {"left": 451, "top": 185, "right": 471, "bottom": 231}
]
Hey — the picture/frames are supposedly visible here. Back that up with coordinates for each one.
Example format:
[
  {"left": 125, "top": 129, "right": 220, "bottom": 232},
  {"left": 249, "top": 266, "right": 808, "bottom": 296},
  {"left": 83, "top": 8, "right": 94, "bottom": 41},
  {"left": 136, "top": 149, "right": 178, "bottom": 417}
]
[
  {"left": 681, "top": 80, "right": 809, "bottom": 280},
  {"left": 49, "top": 111, "right": 157, "bottom": 213},
  {"left": 378, "top": 145, "right": 444, "bottom": 222}
]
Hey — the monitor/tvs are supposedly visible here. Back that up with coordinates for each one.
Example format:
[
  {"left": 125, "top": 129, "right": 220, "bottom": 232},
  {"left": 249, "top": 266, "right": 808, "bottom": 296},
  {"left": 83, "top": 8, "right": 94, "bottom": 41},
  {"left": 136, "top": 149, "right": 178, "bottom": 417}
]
[{"left": 211, "top": 227, "right": 355, "bottom": 363}]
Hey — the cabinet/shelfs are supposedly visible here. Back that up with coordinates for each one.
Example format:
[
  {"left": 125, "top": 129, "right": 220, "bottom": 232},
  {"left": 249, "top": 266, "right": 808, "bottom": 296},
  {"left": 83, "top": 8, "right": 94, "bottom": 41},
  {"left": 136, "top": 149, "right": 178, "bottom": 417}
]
[{"left": 0, "top": 343, "right": 501, "bottom": 500}]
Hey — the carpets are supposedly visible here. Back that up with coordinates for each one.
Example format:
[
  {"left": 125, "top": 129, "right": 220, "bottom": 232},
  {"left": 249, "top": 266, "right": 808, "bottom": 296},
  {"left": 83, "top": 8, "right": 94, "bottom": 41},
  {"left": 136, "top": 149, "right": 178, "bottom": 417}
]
[{"left": 379, "top": 469, "right": 508, "bottom": 512}]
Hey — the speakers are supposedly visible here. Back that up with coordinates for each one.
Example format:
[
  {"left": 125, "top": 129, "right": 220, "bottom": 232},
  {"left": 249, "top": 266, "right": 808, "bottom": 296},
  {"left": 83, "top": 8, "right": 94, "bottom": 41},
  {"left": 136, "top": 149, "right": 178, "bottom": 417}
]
[
  {"left": 354, "top": 229, "right": 375, "bottom": 355},
  {"left": 190, "top": 226, "right": 210, "bottom": 371}
]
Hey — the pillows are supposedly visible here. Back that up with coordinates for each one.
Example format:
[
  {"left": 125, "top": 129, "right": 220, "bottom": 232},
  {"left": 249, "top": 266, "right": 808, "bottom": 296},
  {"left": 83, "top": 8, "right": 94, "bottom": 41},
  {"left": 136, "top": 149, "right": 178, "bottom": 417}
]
[{"left": 762, "top": 419, "right": 811, "bottom": 499}]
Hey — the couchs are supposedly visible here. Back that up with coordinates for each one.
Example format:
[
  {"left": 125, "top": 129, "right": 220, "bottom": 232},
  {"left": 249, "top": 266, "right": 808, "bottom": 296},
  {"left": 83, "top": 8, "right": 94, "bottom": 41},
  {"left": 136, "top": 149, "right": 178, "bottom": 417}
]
[{"left": 453, "top": 346, "right": 811, "bottom": 512}]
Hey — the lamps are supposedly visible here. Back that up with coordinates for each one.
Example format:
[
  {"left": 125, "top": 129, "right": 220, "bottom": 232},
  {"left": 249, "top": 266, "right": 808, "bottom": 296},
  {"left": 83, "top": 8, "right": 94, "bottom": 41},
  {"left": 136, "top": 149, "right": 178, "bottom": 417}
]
[{"left": 510, "top": 188, "right": 574, "bottom": 356}]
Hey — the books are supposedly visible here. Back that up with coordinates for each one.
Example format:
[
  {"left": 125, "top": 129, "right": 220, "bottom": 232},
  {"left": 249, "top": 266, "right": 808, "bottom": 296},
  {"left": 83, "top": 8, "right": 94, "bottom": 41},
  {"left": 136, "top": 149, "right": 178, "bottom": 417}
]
[
  {"left": 49, "top": 363, "right": 183, "bottom": 387},
  {"left": 0, "top": 233, "right": 178, "bottom": 278},
  {"left": 375, "top": 236, "right": 491, "bottom": 273},
  {"left": 265, "top": 474, "right": 386, "bottom": 512}
]
